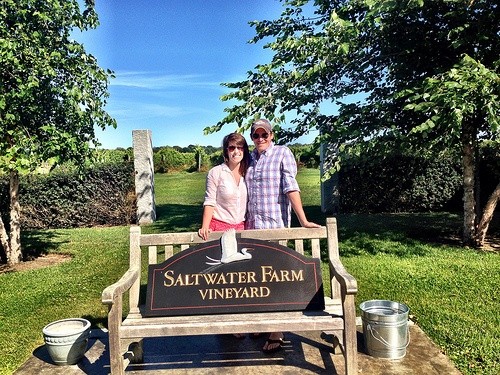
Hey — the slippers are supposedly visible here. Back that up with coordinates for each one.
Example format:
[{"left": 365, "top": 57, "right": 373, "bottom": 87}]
[{"left": 262, "top": 334, "right": 284, "bottom": 352}]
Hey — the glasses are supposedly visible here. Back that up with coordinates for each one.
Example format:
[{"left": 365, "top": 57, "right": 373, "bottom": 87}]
[
  {"left": 253, "top": 133, "right": 270, "bottom": 139},
  {"left": 227, "top": 146, "right": 244, "bottom": 151}
]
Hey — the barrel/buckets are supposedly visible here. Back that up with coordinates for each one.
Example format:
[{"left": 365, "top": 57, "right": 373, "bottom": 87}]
[
  {"left": 359, "top": 300, "right": 410, "bottom": 358},
  {"left": 42, "top": 317, "right": 92, "bottom": 366}
]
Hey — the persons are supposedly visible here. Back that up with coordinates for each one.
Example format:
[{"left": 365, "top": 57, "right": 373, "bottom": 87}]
[
  {"left": 223, "top": 119, "right": 323, "bottom": 353},
  {"left": 198, "top": 133, "right": 255, "bottom": 340}
]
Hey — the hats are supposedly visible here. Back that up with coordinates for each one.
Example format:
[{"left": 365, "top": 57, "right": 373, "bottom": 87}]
[{"left": 251, "top": 119, "right": 271, "bottom": 134}]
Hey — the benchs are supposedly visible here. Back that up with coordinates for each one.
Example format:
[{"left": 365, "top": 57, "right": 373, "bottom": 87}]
[{"left": 102, "top": 217, "right": 358, "bottom": 375}]
